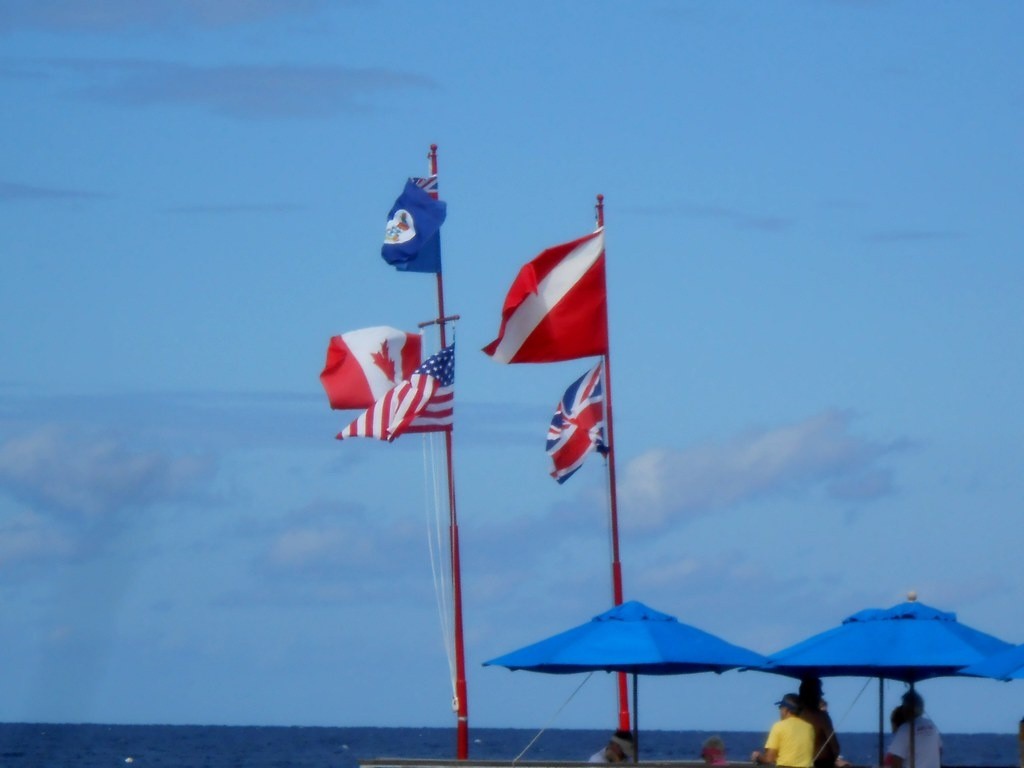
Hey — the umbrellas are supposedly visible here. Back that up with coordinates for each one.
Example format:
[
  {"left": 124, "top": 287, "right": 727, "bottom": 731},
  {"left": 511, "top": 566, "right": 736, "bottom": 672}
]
[
  {"left": 481, "top": 600, "right": 766, "bottom": 763},
  {"left": 738, "top": 592, "right": 1024, "bottom": 768}
]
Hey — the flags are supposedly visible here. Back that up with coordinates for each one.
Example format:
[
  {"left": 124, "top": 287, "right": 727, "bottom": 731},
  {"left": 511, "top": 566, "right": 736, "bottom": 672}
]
[
  {"left": 320, "top": 324, "right": 422, "bottom": 409},
  {"left": 380, "top": 173, "right": 447, "bottom": 273},
  {"left": 480, "top": 225, "right": 608, "bottom": 365},
  {"left": 546, "top": 361, "right": 610, "bottom": 484},
  {"left": 335, "top": 340, "right": 454, "bottom": 443}
]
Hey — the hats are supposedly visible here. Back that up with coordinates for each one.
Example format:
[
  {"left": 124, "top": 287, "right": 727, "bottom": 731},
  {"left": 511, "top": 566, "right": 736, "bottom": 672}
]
[
  {"left": 774, "top": 693, "right": 801, "bottom": 715},
  {"left": 611, "top": 735, "right": 634, "bottom": 758}
]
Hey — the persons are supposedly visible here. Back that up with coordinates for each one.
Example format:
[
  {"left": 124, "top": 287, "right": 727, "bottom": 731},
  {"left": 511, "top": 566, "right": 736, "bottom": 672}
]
[
  {"left": 884, "top": 689, "right": 943, "bottom": 768},
  {"left": 589, "top": 727, "right": 635, "bottom": 763},
  {"left": 701, "top": 736, "right": 727, "bottom": 766},
  {"left": 751, "top": 673, "right": 849, "bottom": 768}
]
